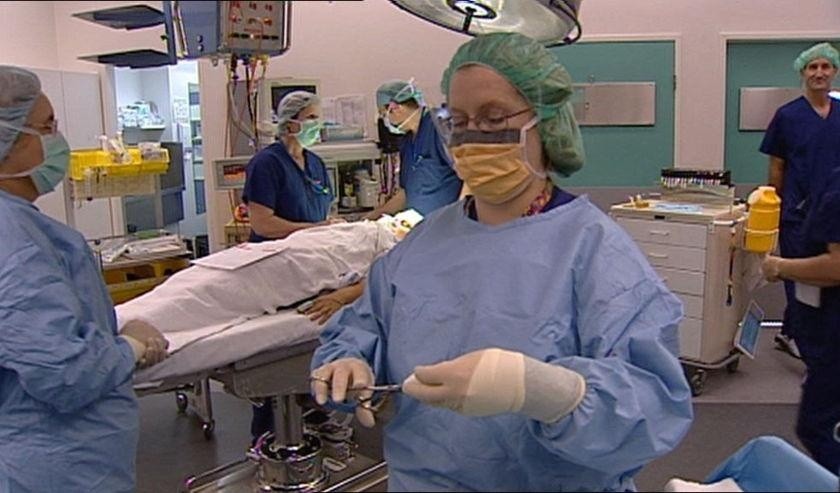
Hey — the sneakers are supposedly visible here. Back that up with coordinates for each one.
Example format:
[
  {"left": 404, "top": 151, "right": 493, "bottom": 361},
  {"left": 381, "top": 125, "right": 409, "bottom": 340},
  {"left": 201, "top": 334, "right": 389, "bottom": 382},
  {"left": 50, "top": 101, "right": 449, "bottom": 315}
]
[
  {"left": 303, "top": 418, "right": 354, "bottom": 441},
  {"left": 774, "top": 333, "right": 801, "bottom": 358}
]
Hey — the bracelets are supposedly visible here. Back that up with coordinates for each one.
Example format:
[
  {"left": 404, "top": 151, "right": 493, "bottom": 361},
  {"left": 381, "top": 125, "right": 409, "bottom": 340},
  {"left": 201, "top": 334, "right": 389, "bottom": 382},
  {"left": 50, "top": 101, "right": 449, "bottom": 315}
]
[{"left": 771, "top": 257, "right": 785, "bottom": 281}]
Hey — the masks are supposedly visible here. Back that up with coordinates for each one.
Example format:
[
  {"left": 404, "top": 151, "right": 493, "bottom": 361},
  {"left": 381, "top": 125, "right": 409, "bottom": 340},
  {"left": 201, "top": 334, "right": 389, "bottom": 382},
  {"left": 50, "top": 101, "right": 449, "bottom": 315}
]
[
  {"left": 293, "top": 118, "right": 322, "bottom": 148},
  {"left": 0, "top": 122, "right": 70, "bottom": 195},
  {"left": 447, "top": 116, "right": 547, "bottom": 205},
  {"left": 383, "top": 113, "right": 409, "bottom": 135}
]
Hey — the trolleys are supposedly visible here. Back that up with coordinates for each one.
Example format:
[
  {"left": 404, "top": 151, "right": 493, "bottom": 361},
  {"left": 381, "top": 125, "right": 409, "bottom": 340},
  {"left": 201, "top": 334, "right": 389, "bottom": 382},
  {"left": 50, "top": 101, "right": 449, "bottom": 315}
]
[{"left": 87, "top": 229, "right": 214, "bottom": 440}]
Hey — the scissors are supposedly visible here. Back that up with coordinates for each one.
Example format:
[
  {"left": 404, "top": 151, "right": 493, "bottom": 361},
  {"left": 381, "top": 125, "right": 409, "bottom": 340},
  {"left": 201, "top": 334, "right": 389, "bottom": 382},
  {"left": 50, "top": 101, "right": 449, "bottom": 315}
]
[{"left": 306, "top": 372, "right": 404, "bottom": 415}]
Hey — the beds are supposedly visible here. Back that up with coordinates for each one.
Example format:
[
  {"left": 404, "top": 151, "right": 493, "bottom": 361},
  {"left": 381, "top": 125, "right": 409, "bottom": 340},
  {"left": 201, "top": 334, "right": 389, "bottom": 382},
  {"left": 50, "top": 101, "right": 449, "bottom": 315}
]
[{"left": 126, "top": 296, "right": 348, "bottom": 400}]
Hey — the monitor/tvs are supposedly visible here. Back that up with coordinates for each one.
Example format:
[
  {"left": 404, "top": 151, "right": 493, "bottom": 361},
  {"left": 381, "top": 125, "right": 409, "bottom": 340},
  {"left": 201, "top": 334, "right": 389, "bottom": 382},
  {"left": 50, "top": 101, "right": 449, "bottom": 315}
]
[{"left": 223, "top": 76, "right": 322, "bottom": 154}]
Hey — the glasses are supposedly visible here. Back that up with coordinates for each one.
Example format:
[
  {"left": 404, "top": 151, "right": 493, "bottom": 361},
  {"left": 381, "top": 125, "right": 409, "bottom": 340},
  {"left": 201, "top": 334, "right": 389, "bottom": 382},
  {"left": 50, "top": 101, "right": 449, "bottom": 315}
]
[
  {"left": 451, "top": 108, "right": 531, "bottom": 133},
  {"left": 23, "top": 120, "right": 58, "bottom": 135}
]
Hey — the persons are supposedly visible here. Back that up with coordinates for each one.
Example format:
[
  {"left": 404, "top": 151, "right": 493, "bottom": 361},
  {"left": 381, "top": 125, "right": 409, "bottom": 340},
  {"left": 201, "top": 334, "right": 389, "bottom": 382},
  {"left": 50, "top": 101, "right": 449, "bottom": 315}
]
[
  {"left": 297, "top": 207, "right": 421, "bottom": 326},
  {"left": 308, "top": 31, "right": 696, "bottom": 492},
  {"left": 241, "top": 89, "right": 355, "bottom": 446},
  {"left": 759, "top": 41, "right": 840, "bottom": 360},
  {"left": 362, "top": 79, "right": 470, "bottom": 227},
  {"left": 761, "top": 166, "right": 840, "bottom": 478},
  {"left": 1, "top": 65, "right": 172, "bottom": 492}
]
